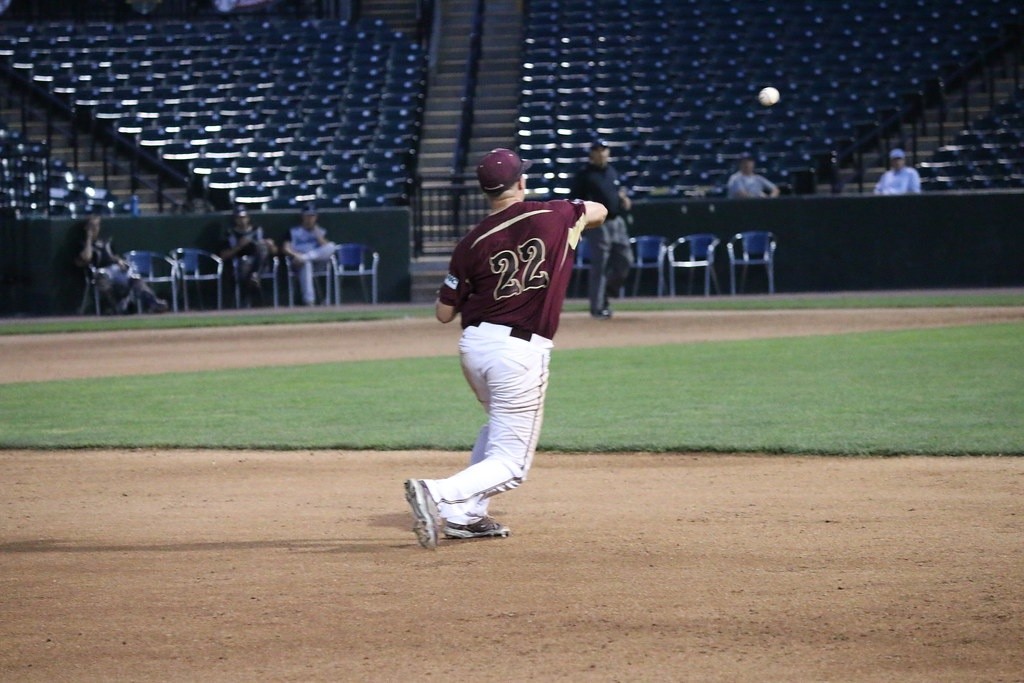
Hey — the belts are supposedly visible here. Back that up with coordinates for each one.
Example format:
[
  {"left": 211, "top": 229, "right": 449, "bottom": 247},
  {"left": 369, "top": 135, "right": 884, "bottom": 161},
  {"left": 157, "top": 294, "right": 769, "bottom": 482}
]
[{"left": 470, "top": 320, "right": 532, "bottom": 342}]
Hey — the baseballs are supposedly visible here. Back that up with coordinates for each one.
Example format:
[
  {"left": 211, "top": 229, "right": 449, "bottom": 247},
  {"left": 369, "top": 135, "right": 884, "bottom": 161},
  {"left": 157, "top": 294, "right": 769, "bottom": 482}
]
[{"left": 758, "top": 86, "right": 780, "bottom": 106}]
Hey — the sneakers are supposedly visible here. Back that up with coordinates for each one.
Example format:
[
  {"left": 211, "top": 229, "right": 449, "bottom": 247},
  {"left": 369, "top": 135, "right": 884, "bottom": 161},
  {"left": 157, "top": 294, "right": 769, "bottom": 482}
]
[
  {"left": 444, "top": 514, "right": 511, "bottom": 539},
  {"left": 404, "top": 478, "right": 438, "bottom": 551}
]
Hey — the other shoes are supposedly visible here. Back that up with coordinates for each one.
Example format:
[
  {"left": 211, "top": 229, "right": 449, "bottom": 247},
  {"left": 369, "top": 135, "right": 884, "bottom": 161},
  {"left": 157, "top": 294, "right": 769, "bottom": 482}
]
[{"left": 593, "top": 309, "right": 610, "bottom": 319}]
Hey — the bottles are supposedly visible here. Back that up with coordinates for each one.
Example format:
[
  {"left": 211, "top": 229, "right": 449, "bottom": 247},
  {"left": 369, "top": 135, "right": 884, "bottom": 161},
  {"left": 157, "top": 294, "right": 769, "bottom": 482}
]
[{"left": 130, "top": 195, "right": 139, "bottom": 217}]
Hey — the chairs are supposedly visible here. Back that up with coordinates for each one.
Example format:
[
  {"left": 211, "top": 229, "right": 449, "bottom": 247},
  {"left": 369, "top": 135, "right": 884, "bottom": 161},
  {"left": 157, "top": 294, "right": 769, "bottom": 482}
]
[
  {"left": 513, "top": 0, "right": 1024, "bottom": 196},
  {"left": 616, "top": 234, "right": 668, "bottom": 298},
  {"left": 331, "top": 243, "right": 381, "bottom": 305},
  {"left": 0, "top": 11, "right": 429, "bottom": 214},
  {"left": 84, "top": 262, "right": 143, "bottom": 316},
  {"left": 285, "top": 253, "right": 331, "bottom": 307},
  {"left": 168, "top": 247, "right": 224, "bottom": 311},
  {"left": 726, "top": 230, "right": 778, "bottom": 295},
  {"left": 122, "top": 250, "right": 181, "bottom": 312},
  {"left": 665, "top": 234, "right": 721, "bottom": 297},
  {"left": 575, "top": 237, "right": 590, "bottom": 294},
  {"left": 234, "top": 256, "right": 279, "bottom": 309}
]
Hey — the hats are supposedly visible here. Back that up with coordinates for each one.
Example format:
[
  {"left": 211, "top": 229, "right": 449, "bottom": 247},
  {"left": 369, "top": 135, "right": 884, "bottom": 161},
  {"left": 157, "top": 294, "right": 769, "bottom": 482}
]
[
  {"left": 476, "top": 148, "right": 533, "bottom": 191},
  {"left": 590, "top": 138, "right": 608, "bottom": 151},
  {"left": 890, "top": 149, "right": 905, "bottom": 159}
]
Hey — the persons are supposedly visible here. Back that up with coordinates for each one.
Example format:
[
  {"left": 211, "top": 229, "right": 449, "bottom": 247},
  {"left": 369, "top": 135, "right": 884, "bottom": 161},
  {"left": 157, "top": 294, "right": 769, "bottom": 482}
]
[
  {"left": 73, "top": 215, "right": 169, "bottom": 314},
  {"left": 218, "top": 204, "right": 279, "bottom": 295},
  {"left": 872, "top": 148, "right": 921, "bottom": 195},
  {"left": 725, "top": 152, "right": 780, "bottom": 201},
  {"left": 280, "top": 202, "right": 337, "bottom": 308},
  {"left": 569, "top": 141, "right": 635, "bottom": 321},
  {"left": 405, "top": 149, "right": 609, "bottom": 553}
]
